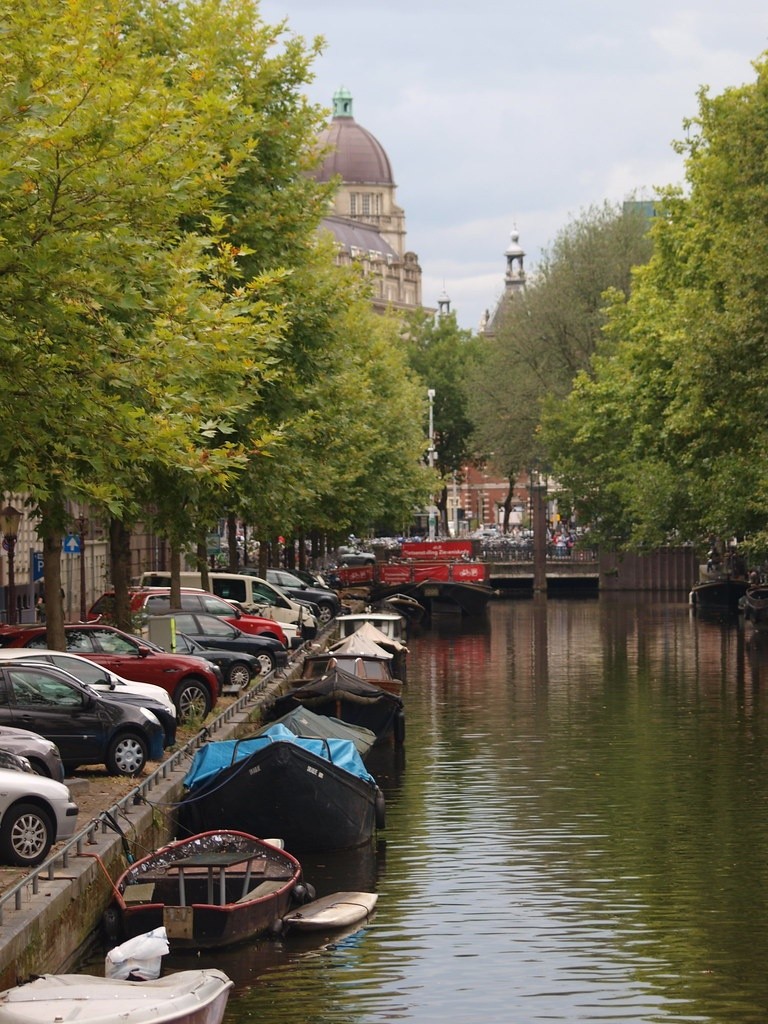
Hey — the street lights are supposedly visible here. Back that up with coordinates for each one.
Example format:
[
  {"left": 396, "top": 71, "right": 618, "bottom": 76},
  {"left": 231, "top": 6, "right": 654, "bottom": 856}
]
[{"left": 0, "top": 499, "right": 24, "bottom": 624}]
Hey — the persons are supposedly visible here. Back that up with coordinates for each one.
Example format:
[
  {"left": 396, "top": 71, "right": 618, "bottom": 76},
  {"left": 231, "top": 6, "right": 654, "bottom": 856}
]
[
  {"left": 37, "top": 579, "right": 67, "bottom": 624},
  {"left": 553, "top": 530, "right": 573, "bottom": 560}
]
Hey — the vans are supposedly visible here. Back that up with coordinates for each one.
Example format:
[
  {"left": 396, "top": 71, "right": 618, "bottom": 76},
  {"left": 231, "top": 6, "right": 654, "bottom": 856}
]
[{"left": 140, "top": 570, "right": 318, "bottom": 640}]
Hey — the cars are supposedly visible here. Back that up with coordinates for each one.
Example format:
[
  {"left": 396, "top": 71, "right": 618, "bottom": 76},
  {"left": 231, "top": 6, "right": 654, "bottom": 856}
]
[
  {"left": 323, "top": 546, "right": 377, "bottom": 570},
  {"left": 233, "top": 569, "right": 340, "bottom": 624},
  {"left": 0, "top": 646, "right": 176, "bottom": 719},
  {"left": 0, "top": 726, "right": 65, "bottom": 782},
  {"left": 386, "top": 525, "right": 593, "bottom": 558},
  {"left": 141, "top": 632, "right": 262, "bottom": 691},
  {"left": 140, "top": 609, "right": 286, "bottom": 677},
  {"left": 0, "top": 768, "right": 79, "bottom": 867},
  {"left": 126, "top": 632, "right": 224, "bottom": 701},
  {"left": 0, "top": 663, "right": 172, "bottom": 777},
  {"left": 0, "top": 748, "right": 33, "bottom": 775},
  {"left": 206, "top": 599, "right": 303, "bottom": 650},
  {"left": 0, "top": 623, "right": 220, "bottom": 724}
]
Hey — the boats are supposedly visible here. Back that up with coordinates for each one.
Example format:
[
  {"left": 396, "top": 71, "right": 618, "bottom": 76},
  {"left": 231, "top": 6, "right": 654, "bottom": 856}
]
[
  {"left": 692, "top": 557, "right": 751, "bottom": 606},
  {"left": 744, "top": 571, "right": 768, "bottom": 623},
  {"left": 111, "top": 829, "right": 303, "bottom": 951},
  {"left": 0, "top": 967, "right": 235, "bottom": 1024},
  {"left": 276, "top": 652, "right": 403, "bottom": 734},
  {"left": 327, "top": 577, "right": 496, "bottom": 674},
  {"left": 178, "top": 704, "right": 386, "bottom": 850}
]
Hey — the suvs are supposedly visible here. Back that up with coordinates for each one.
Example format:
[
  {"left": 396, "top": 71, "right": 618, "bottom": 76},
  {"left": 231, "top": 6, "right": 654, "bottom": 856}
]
[{"left": 86, "top": 591, "right": 287, "bottom": 646}]
